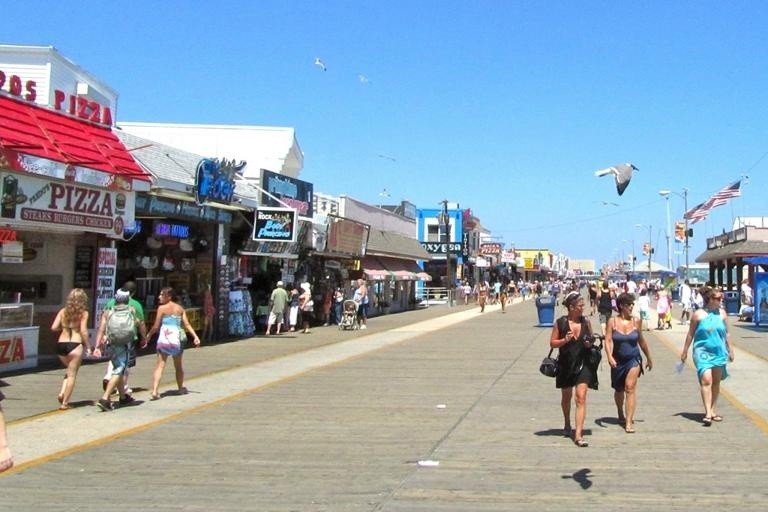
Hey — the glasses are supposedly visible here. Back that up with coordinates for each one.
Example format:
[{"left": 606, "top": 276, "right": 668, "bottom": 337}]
[{"left": 713, "top": 294, "right": 723, "bottom": 300}]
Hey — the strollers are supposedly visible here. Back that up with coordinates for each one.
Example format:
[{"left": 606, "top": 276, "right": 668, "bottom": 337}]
[{"left": 338, "top": 298, "right": 361, "bottom": 332}]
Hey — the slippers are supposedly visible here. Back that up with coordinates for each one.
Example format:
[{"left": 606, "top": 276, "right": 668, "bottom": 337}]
[
  {"left": 702, "top": 416, "right": 712, "bottom": 424},
  {"left": 626, "top": 427, "right": 634, "bottom": 433},
  {"left": 712, "top": 415, "right": 723, "bottom": 420},
  {"left": 575, "top": 438, "right": 588, "bottom": 446}
]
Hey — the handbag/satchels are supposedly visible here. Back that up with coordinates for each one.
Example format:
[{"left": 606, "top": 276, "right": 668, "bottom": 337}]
[
  {"left": 303, "top": 299, "right": 314, "bottom": 312},
  {"left": 132, "top": 235, "right": 209, "bottom": 272},
  {"left": 540, "top": 358, "right": 559, "bottom": 377},
  {"left": 585, "top": 344, "right": 601, "bottom": 375}
]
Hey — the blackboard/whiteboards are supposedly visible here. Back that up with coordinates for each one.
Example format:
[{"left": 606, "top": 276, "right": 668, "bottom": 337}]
[{"left": 73, "top": 245, "right": 94, "bottom": 288}]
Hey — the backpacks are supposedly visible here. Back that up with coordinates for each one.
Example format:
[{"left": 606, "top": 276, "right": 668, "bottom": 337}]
[{"left": 106, "top": 305, "right": 136, "bottom": 347}]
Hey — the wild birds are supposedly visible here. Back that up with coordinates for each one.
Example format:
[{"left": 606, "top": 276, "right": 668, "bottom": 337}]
[
  {"left": 314, "top": 57, "right": 326, "bottom": 72},
  {"left": 379, "top": 188, "right": 392, "bottom": 200},
  {"left": 378, "top": 154, "right": 396, "bottom": 164},
  {"left": 591, "top": 200, "right": 621, "bottom": 207},
  {"left": 357, "top": 74, "right": 371, "bottom": 86},
  {"left": 594, "top": 162, "right": 640, "bottom": 197}
]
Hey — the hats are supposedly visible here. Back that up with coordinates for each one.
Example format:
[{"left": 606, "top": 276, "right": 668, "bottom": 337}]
[
  {"left": 301, "top": 282, "right": 312, "bottom": 294},
  {"left": 115, "top": 288, "right": 130, "bottom": 303},
  {"left": 561, "top": 290, "right": 583, "bottom": 306},
  {"left": 276, "top": 281, "right": 284, "bottom": 286}
]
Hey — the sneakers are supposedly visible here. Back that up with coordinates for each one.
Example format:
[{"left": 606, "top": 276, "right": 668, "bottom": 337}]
[{"left": 97, "top": 378, "right": 135, "bottom": 412}]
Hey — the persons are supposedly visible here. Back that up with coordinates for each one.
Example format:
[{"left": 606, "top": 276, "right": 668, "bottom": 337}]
[
  {"left": 461, "top": 274, "right": 756, "bottom": 339},
  {"left": 265, "top": 278, "right": 369, "bottom": 335},
  {"left": 100, "top": 281, "right": 148, "bottom": 394},
  {"left": 0, "top": 404, "right": 13, "bottom": 472},
  {"left": 681, "top": 287, "right": 735, "bottom": 424},
  {"left": 604, "top": 293, "right": 653, "bottom": 433},
  {"left": 51, "top": 288, "right": 92, "bottom": 409},
  {"left": 92, "top": 287, "right": 140, "bottom": 412},
  {"left": 550, "top": 292, "right": 598, "bottom": 448},
  {"left": 146, "top": 286, "right": 201, "bottom": 399}
]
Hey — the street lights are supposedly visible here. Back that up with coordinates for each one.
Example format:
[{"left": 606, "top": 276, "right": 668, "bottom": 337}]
[
  {"left": 603, "top": 238, "right": 635, "bottom": 280},
  {"left": 634, "top": 223, "right": 652, "bottom": 276},
  {"left": 658, "top": 186, "right": 689, "bottom": 279}
]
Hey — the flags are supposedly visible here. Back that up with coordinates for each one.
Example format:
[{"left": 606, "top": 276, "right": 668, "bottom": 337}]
[{"left": 683, "top": 181, "right": 740, "bottom": 225}]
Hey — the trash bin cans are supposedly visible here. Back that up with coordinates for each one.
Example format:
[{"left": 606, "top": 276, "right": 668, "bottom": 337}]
[
  {"left": 724, "top": 291, "right": 740, "bottom": 316},
  {"left": 671, "top": 287, "right": 679, "bottom": 300},
  {"left": 533, "top": 296, "right": 556, "bottom": 327}
]
